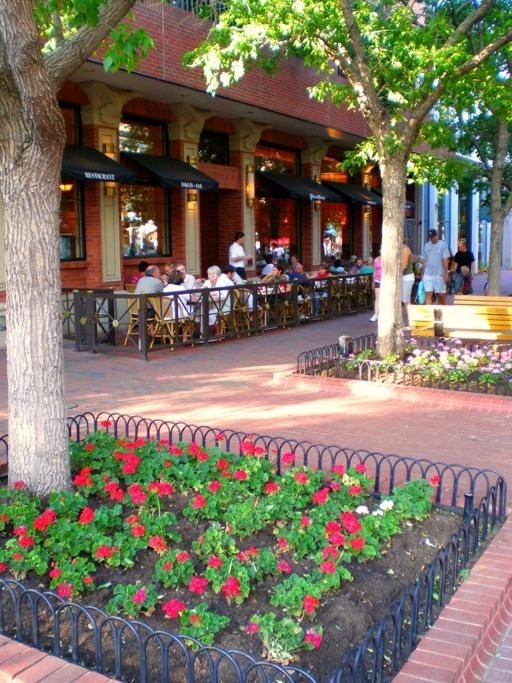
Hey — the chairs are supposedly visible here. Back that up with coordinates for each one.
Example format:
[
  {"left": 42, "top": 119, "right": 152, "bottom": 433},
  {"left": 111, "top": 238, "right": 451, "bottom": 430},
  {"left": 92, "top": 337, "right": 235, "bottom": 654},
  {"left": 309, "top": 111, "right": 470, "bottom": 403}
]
[{"left": 124, "top": 268, "right": 376, "bottom": 353}]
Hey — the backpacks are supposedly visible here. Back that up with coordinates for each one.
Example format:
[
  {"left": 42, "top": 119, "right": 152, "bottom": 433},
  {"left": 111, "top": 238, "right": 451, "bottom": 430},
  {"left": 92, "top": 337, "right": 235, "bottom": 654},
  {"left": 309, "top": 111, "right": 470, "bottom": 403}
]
[{"left": 459, "top": 275, "right": 473, "bottom": 295}]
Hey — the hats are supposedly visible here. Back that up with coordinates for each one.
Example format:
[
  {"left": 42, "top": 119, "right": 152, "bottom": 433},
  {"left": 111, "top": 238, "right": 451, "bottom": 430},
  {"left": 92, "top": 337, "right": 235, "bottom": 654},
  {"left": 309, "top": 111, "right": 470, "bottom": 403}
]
[{"left": 427, "top": 228, "right": 436, "bottom": 237}]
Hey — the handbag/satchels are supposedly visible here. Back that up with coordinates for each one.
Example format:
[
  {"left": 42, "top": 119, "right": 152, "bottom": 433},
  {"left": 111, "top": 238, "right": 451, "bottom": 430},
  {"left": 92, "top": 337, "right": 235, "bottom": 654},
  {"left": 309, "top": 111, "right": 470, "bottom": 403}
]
[{"left": 417, "top": 280, "right": 428, "bottom": 304}]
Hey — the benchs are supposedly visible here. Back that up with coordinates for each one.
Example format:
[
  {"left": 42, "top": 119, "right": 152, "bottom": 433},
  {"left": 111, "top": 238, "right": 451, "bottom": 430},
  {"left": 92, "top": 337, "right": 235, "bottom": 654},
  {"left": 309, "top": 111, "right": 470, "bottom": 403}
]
[{"left": 407, "top": 294, "right": 512, "bottom": 343}]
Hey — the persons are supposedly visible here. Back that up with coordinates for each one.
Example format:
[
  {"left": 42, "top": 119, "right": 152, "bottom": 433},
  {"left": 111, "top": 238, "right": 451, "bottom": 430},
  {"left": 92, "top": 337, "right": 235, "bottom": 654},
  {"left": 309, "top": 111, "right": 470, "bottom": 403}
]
[
  {"left": 122, "top": 231, "right": 383, "bottom": 350},
  {"left": 400, "top": 233, "right": 417, "bottom": 332},
  {"left": 419, "top": 228, "right": 452, "bottom": 305},
  {"left": 449, "top": 237, "right": 476, "bottom": 294}
]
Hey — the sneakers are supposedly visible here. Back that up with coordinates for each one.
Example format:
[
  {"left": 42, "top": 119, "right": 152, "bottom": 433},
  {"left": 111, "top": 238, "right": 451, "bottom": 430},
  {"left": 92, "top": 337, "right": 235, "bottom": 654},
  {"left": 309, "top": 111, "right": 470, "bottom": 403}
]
[
  {"left": 368, "top": 314, "right": 377, "bottom": 322},
  {"left": 297, "top": 313, "right": 321, "bottom": 323}
]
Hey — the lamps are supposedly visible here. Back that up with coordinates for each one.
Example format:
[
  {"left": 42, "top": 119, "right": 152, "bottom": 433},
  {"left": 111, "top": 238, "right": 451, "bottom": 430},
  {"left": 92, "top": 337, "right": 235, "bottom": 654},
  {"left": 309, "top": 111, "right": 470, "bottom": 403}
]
[
  {"left": 187, "top": 155, "right": 199, "bottom": 202},
  {"left": 313, "top": 174, "right": 322, "bottom": 210},
  {"left": 102, "top": 140, "right": 116, "bottom": 196},
  {"left": 245, "top": 164, "right": 256, "bottom": 207}
]
